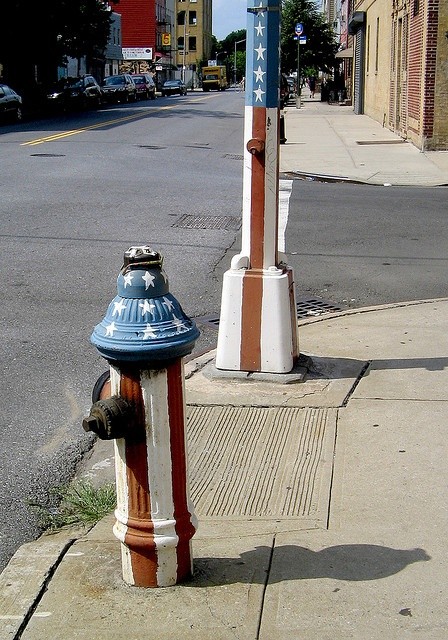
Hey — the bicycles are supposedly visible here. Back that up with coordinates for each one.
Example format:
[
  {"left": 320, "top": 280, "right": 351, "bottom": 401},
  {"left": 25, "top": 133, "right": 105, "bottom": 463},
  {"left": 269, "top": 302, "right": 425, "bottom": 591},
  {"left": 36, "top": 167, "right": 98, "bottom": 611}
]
[{"left": 234, "top": 79, "right": 244, "bottom": 93}]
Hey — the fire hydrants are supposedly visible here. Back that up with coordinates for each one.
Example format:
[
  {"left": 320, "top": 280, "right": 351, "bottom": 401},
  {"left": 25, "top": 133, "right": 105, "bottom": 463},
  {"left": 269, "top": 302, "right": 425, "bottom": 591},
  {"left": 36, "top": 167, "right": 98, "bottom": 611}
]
[{"left": 82, "top": 245, "right": 202, "bottom": 587}]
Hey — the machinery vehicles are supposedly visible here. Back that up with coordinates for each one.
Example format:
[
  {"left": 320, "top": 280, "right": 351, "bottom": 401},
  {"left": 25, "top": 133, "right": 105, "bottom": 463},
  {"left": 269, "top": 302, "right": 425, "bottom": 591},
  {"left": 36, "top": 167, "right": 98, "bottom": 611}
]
[{"left": 201, "top": 65, "right": 227, "bottom": 91}]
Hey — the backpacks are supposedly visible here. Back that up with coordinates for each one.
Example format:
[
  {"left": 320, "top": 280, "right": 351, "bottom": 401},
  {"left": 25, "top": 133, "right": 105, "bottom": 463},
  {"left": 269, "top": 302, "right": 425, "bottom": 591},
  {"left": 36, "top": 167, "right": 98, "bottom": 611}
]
[{"left": 310, "top": 82, "right": 316, "bottom": 91}]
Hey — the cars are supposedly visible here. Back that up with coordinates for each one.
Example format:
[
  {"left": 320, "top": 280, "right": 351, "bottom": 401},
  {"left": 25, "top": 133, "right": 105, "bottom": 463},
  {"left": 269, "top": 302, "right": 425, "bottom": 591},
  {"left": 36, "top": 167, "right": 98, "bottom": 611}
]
[
  {"left": 161, "top": 79, "right": 187, "bottom": 97},
  {"left": 43, "top": 73, "right": 102, "bottom": 110},
  {"left": 129, "top": 74, "right": 157, "bottom": 99},
  {"left": 100, "top": 74, "right": 137, "bottom": 104},
  {"left": 0, "top": 84, "right": 23, "bottom": 122}
]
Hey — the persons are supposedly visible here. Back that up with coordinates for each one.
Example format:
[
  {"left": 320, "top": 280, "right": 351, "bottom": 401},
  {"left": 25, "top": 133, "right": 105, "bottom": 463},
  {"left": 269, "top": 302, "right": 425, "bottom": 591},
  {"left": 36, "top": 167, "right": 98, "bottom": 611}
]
[
  {"left": 242, "top": 76, "right": 246, "bottom": 91},
  {"left": 309, "top": 74, "right": 318, "bottom": 99}
]
